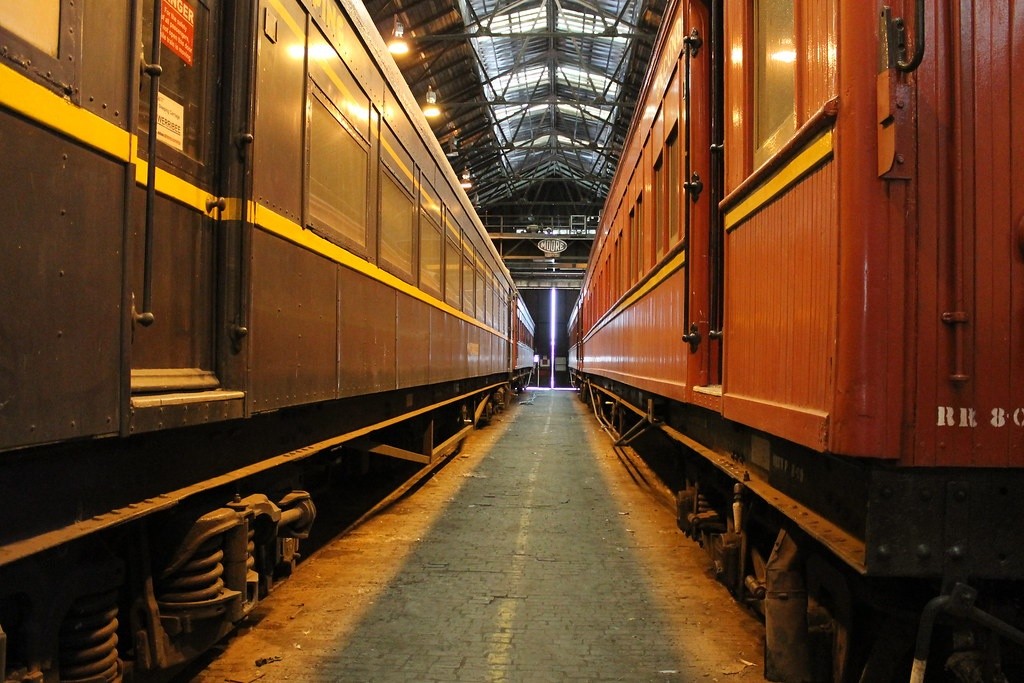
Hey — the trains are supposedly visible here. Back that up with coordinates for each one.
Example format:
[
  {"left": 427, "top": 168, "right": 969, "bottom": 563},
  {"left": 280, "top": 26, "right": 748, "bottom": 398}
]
[
  {"left": 568, "top": 0, "right": 1024, "bottom": 683},
  {"left": 0, "top": 1, "right": 537, "bottom": 683}
]
